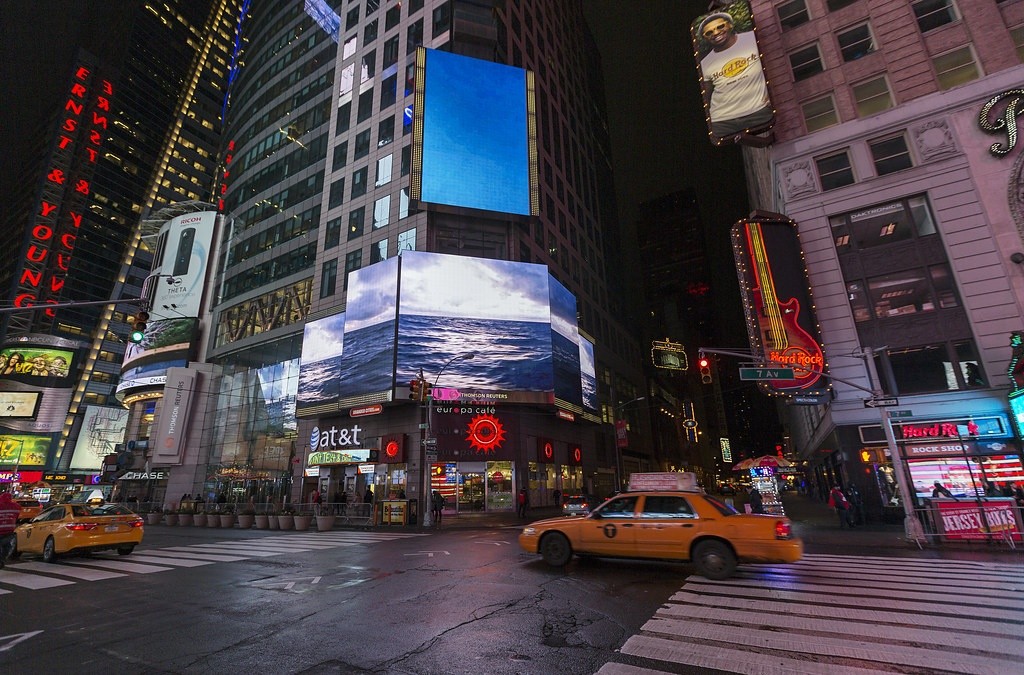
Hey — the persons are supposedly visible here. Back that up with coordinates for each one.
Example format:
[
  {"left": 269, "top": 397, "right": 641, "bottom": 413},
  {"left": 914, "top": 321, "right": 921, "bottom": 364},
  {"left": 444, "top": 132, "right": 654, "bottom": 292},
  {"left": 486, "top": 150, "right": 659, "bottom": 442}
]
[
  {"left": 186, "top": 494, "right": 193, "bottom": 500},
  {"left": 932, "top": 480, "right": 952, "bottom": 497},
  {"left": 828, "top": 480, "right": 853, "bottom": 527},
  {"left": 312, "top": 489, "right": 322, "bottom": 522},
  {"left": 433, "top": 490, "right": 445, "bottom": 523},
  {"left": 179, "top": 493, "right": 187, "bottom": 511},
  {"left": 846, "top": 479, "right": 862, "bottom": 526},
  {"left": 1004, "top": 480, "right": 1024, "bottom": 506},
  {"left": 332, "top": 492, "right": 347, "bottom": 516},
  {"left": 981, "top": 479, "right": 1001, "bottom": 497},
  {"left": 553, "top": 487, "right": 561, "bottom": 506},
  {"left": 363, "top": 485, "right": 374, "bottom": 517},
  {"left": 395, "top": 489, "right": 406, "bottom": 499},
  {"left": 746, "top": 486, "right": 763, "bottom": 513},
  {"left": 518, "top": 487, "right": 529, "bottom": 519},
  {"left": 195, "top": 494, "right": 201, "bottom": 501},
  {"left": 105, "top": 494, "right": 111, "bottom": 503}
]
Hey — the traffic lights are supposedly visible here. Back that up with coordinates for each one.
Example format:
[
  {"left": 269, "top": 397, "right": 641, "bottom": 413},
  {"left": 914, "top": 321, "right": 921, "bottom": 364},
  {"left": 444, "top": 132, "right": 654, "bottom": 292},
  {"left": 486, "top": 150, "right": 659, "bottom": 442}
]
[
  {"left": 128, "top": 311, "right": 150, "bottom": 343},
  {"left": 698, "top": 358, "right": 712, "bottom": 385},
  {"left": 422, "top": 381, "right": 433, "bottom": 402},
  {"left": 408, "top": 380, "right": 420, "bottom": 401}
]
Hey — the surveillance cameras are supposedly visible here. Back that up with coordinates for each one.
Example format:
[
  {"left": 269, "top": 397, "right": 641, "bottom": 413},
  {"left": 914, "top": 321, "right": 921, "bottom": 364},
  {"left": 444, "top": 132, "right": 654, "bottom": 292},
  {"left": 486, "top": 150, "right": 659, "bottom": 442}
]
[{"left": 167, "top": 278, "right": 174, "bottom": 285}]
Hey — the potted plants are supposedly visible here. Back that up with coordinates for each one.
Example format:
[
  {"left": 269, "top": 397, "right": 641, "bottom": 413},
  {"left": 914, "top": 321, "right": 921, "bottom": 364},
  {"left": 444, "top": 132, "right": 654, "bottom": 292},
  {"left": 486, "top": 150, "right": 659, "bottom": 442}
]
[
  {"left": 294, "top": 511, "right": 312, "bottom": 531},
  {"left": 254, "top": 510, "right": 294, "bottom": 530},
  {"left": 316, "top": 506, "right": 337, "bottom": 532},
  {"left": 163, "top": 507, "right": 237, "bottom": 529},
  {"left": 238, "top": 508, "right": 256, "bottom": 528},
  {"left": 147, "top": 506, "right": 163, "bottom": 526}
]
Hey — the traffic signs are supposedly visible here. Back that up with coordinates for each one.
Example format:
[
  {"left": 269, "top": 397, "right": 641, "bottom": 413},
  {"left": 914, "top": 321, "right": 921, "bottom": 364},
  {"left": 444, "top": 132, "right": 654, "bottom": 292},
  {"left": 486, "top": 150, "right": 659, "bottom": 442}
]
[
  {"left": 863, "top": 398, "right": 899, "bottom": 409},
  {"left": 422, "top": 438, "right": 437, "bottom": 451}
]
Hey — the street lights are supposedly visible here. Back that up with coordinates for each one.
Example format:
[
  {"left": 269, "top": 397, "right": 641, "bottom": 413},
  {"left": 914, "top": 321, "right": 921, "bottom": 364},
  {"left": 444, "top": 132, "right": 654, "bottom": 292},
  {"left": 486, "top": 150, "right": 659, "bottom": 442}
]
[
  {"left": 423, "top": 352, "right": 475, "bottom": 526},
  {"left": 0, "top": 436, "right": 24, "bottom": 493}
]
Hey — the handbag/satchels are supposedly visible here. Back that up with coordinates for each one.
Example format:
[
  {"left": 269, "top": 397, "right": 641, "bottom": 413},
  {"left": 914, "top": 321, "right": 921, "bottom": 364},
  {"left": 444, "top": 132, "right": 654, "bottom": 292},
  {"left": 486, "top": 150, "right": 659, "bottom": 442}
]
[{"left": 318, "top": 496, "right": 322, "bottom": 503}]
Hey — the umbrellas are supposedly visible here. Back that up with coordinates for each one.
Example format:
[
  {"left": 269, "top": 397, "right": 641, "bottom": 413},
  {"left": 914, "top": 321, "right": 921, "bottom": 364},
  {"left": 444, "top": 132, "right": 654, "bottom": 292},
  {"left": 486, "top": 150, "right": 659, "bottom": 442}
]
[
  {"left": 748, "top": 455, "right": 794, "bottom": 471},
  {"left": 732, "top": 458, "right": 756, "bottom": 471}
]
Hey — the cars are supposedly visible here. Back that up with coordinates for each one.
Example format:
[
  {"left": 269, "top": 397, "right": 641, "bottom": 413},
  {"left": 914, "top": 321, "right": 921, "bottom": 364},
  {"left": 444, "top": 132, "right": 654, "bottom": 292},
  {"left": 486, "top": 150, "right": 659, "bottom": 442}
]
[
  {"left": 10, "top": 502, "right": 144, "bottom": 564},
  {"left": 719, "top": 483, "right": 737, "bottom": 495},
  {"left": 14, "top": 499, "right": 44, "bottom": 524},
  {"left": 519, "top": 471, "right": 803, "bottom": 581}
]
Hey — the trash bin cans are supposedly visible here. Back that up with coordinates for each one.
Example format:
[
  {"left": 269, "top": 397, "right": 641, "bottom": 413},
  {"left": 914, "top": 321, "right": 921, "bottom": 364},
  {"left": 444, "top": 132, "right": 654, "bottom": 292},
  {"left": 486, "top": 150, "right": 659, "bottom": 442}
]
[
  {"left": 179, "top": 500, "right": 205, "bottom": 512},
  {"left": 381, "top": 498, "right": 417, "bottom": 525}
]
